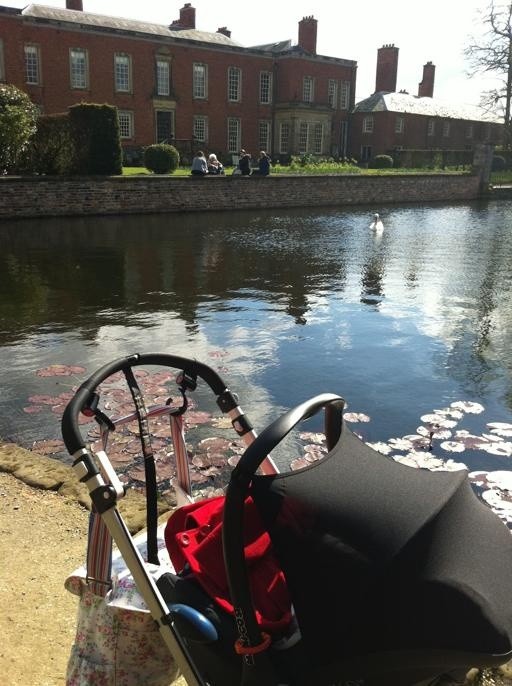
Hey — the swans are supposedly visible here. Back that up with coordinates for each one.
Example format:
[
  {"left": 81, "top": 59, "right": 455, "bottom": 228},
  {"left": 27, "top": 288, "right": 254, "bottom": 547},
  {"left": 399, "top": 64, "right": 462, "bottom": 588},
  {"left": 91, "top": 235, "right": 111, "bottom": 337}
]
[{"left": 368, "top": 212, "right": 385, "bottom": 235}]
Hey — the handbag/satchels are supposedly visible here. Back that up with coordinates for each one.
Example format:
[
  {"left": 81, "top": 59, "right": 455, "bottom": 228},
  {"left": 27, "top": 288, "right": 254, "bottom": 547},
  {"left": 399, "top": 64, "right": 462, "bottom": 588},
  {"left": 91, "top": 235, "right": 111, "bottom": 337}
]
[{"left": 65, "top": 501, "right": 190, "bottom": 686}]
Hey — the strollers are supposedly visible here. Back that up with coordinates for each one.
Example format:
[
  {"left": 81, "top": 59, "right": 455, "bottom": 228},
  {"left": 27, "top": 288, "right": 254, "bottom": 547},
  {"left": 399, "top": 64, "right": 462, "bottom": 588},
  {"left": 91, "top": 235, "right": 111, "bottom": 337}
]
[{"left": 60, "top": 348, "right": 511, "bottom": 686}]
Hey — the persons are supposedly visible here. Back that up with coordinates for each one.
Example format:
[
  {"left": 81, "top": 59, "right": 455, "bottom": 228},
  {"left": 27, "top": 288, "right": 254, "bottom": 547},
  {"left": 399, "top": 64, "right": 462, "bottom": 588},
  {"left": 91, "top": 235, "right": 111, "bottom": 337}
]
[
  {"left": 251, "top": 151, "right": 270, "bottom": 175},
  {"left": 207, "top": 154, "right": 224, "bottom": 175},
  {"left": 191, "top": 151, "right": 208, "bottom": 175},
  {"left": 232, "top": 149, "right": 251, "bottom": 176}
]
